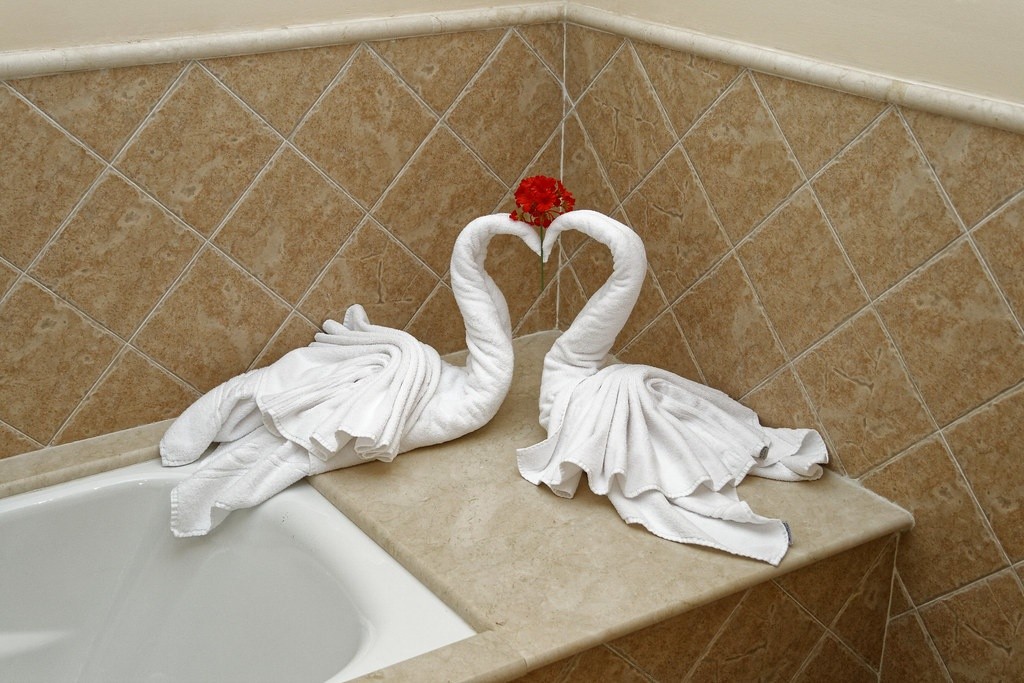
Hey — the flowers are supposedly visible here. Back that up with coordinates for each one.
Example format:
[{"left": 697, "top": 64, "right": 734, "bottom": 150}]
[{"left": 506, "top": 173, "right": 577, "bottom": 294}]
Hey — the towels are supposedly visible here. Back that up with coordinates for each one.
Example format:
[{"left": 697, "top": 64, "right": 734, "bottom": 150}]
[{"left": 157, "top": 209, "right": 831, "bottom": 568}]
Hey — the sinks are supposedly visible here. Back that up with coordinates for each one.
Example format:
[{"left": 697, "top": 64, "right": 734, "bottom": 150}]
[{"left": 0, "top": 437, "right": 480, "bottom": 682}]
